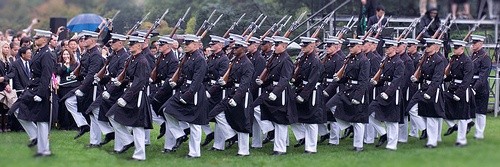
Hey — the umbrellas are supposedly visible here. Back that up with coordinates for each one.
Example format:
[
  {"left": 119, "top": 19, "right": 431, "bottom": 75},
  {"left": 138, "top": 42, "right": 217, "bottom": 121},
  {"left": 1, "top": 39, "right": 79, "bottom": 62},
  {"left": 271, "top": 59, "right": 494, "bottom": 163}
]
[{"left": 66, "top": 14, "right": 108, "bottom": 33}]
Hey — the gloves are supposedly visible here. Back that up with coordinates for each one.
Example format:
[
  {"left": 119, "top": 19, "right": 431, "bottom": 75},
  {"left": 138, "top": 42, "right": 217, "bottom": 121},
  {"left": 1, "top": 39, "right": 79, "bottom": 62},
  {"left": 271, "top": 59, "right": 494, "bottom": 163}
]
[
  {"left": 34, "top": 94, "right": 42, "bottom": 101},
  {"left": 352, "top": 99, "right": 360, "bottom": 105},
  {"left": 228, "top": 99, "right": 237, "bottom": 107},
  {"left": 179, "top": 96, "right": 186, "bottom": 104},
  {"left": 94, "top": 73, "right": 100, "bottom": 82},
  {"left": 75, "top": 89, "right": 83, "bottom": 97},
  {"left": 381, "top": 92, "right": 388, "bottom": 100},
  {"left": 102, "top": 91, "right": 111, "bottom": 100},
  {"left": 269, "top": 92, "right": 277, "bottom": 101},
  {"left": 114, "top": 77, "right": 122, "bottom": 86},
  {"left": 371, "top": 78, "right": 377, "bottom": 86},
  {"left": 256, "top": 76, "right": 263, "bottom": 85},
  {"left": 70, "top": 72, "right": 77, "bottom": 79},
  {"left": 296, "top": 95, "right": 305, "bottom": 102},
  {"left": 444, "top": 75, "right": 447, "bottom": 79},
  {"left": 410, "top": 75, "right": 418, "bottom": 82},
  {"left": 218, "top": 77, "right": 228, "bottom": 87},
  {"left": 149, "top": 78, "right": 153, "bottom": 84},
  {"left": 292, "top": 77, "right": 296, "bottom": 82},
  {"left": 453, "top": 94, "right": 461, "bottom": 101},
  {"left": 206, "top": 90, "right": 211, "bottom": 98},
  {"left": 333, "top": 73, "right": 341, "bottom": 82},
  {"left": 321, "top": 90, "right": 329, "bottom": 97},
  {"left": 472, "top": 89, "right": 476, "bottom": 96},
  {"left": 117, "top": 97, "right": 127, "bottom": 107},
  {"left": 169, "top": 79, "right": 177, "bottom": 88},
  {"left": 424, "top": 93, "right": 431, "bottom": 100}
]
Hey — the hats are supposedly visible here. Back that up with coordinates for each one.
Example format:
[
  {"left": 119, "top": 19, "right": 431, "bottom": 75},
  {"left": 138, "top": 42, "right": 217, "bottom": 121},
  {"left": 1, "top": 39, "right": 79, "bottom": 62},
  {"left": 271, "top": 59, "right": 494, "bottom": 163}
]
[
  {"left": 365, "top": 35, "right": 380, "bottom": 44},
  {"left": 136, "top": 31, "right": 153, "bottom": 39},
  {"left": 347, "top": 39, "right": 364, "bottom": 46},
  {"left": 424, "top": 38, "right": 438, "bottom": 47},
  {"left": 82, "top": 30, "right": 100, "bottom": 39},
  {"left": 232, "top": 40, "right": 250, "bottom": 48},
  {"left": 260, "top": 36, "right": 274, "bottom": 43},
  {"left": 436, "top": 39, "right": 444, "bottom": 45},
  {"left": 185, "top": 35, "right": 199, "bottom": 44},
  {"left": 246, "top": 35, "right": 262, "bottom": 44},
  {"left": 33, "top": 29, "right": 52, "bottom": 39},
  {"left": 400, "top": 38, "right": 407, "bottom": 43},
  {"left": 229, "top": 33, "right": 244, "bottom": 41},
  {"left": 127, "top": 36, "right": 145, "bottom": 44},
  {"left": 452, "top": 40, "right": 467, "bottom": 47},
  {"left": 300, "top": 37, "right": 318, "bottom": 46},
  {"left": 358, "top": 35, "right": 373, "bottom": 43},
  {"left": 382, "top": 39, "right": 400, "bottom": 47},
  {"left": 471, "top": 34, "right": 486, "bottom": 43},
  {"left": 209, "top": 35, "right": 225, "bottom": 45},
  {"left": 157, "top": 37, "right": 174, "bottom": 44},
  {"left": 110, "top": 33, "right": 128, "bottom": 42},
  {"left": 272, "top": 36, "right": 290, "bottom": 45},
  {"left": 407, "top": 38, "right": 420, "bottom": 46},
  {"left": 330, "top": 35, "right": 345, "bottom": 44},
  {"left": 325, "top": 39, "right": 338, "bottom": 46}
]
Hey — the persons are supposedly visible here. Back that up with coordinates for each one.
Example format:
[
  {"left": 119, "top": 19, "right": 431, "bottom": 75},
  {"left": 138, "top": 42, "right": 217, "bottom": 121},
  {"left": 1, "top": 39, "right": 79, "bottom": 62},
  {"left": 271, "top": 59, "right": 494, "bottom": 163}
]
[
  {"left": 245, "top": 37, "right": 266, "bottom": 150},
  {"left": 416, "top": 7, "right": 441, "bottom": 43},
  {"left": 451, "top": 0, "right": 470, "bottom": 20},
  {"left": 466, "top": 35, "right": 491, "bottom": 139},
  {"left": 254, "top": 36, "right": 294, "bottom": 155},
  {"left": 163, "top": 35, "right": 207, "bottom": 159},
  {"left": 92, "top": 34, "right": 129, "bottom": 144},
  {"left": 0, "top": 19, "right": 385, "bottom": 131},
  {"left": 366, "top": 6, "right": 389, "bottom": 39},
  {"left": 318, "top": 39, "right": 344, "bottom": 146},
  {"left": 290, "top": 37, "right": 326, "bottom": 154},
  {"left": 409, "top": 38, "right": 444, "bottom": 148},
  {"left": 150, "top": 35, "right": 179, "bottom": 152},
  {"left": 328, "top": 38, "right": 370, "bottom": 152},
  {"left": 433, "top": 39, "right": 448, "bottom": 77},
  {"left": 201, "top": 36, "right": 229, "bottom": 151},
  {"left": 369, "top": 39, "right": 405, "bottom": 150},
  {"left": 443, "top": 39, "right": 473, "bottom": 147},
  {"left": 406, "top": 38, "right": 421, "bottom": 137},
  {"left": 214, "top": 39, "right": 254, "bottom": 157},
  {"left": 108, "top": 36, "right": 151, "bottom": 161},
  {"left": 419, "top": 0, "right": 437, "bottom": 17},
  {"left": 356, "top": 0, "right": 377, "bottom": 38},
  {"left": 341, "top": 35, "right": 380, "bottom": 144},
  {"left": 65, "top": 31, "right": 103, "bottom": 149},
  {"left": 393, "top": 38, "right": 415, "bottom": 144},
  {"left": 12, "top": 30, "right": 56, "bottom": 158}
]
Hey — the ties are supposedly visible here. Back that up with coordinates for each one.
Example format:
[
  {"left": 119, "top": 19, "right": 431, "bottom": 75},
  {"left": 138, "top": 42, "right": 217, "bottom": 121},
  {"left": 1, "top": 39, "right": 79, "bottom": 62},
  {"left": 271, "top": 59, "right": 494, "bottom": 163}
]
[{"left": 25, "top": 62, "right": 29, "bottom": 76}]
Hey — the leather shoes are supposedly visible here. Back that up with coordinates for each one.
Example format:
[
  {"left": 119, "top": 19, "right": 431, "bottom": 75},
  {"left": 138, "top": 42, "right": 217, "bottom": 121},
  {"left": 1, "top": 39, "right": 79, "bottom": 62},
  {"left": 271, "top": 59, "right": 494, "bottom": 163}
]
[
  {"left": 202, "top": 132, "right": 214, "bottom": 146},
  {"left": 34, "top": 154, "right": 50, "bottom": 158},
  {"left": 74, "top": 125, "right": 90, "bottom": 140},
  {"left": 118, "top": 141, "right": 134, "bottom": 154},
  {"left": 425, "top": 145, "right": 435, "bottom": 148},
  {"left": 455, "top": 142, "right": 463, "bottom": 147},
  {"left": 319, "top": 133, "right": 329, "bottom": 143},
  {"left": 467, "top": 122, "right": 475, "bottom": 133},
  {"left": 263, "top": 130, "right": 275, "bottom": 144},
  {"left": 227, "top": 140, "right": 233, "bottom": 147},
  {"left": 304, "top": 151, "right": 315, "bottom": 155},
  {"left": 235, "top": 154, "right": 245, "bottom": 157},
  {"left": 420, "top": 129, "right": 427, "bottom": 140},
  {"left": 270, "top": 151, "right": 285, "bottom": 156},
  {"left": 127, "top": 158, "right": 137, "bottom": 161},
  {"left": 182, "top": 155, "right": 194, "bottom": 159},
  {"left": 27, "top": 139, "right": 38, "bottom": 147},
  {"left": 353, "top": 147, "right": 363, "bottom": 151},
  {"left": 377, "top": 134, "right": 387, "bottom": 146},
  {"left": 157, "top": 125, "right": 166, "bottom": 139},
  {"left": 341, "top": 125, "right": 353, "bottom": 140},
  {"left": 294, "top": 138, "right": 305, "bottom": 147},
  {"left": 100, "top": 132, "right": 114, "bottom": 145},
  {"left": 87, "top": 144, "right": 101, "bottom": 148},
  {"left": 172, "top": 135, "right": 188, "bottom": 149},
  {"left": 209, "top": 147, "right": 223, "bottom": 152},
  {"left": 444, "top": 124, "right": 458, "bottom": 136}
]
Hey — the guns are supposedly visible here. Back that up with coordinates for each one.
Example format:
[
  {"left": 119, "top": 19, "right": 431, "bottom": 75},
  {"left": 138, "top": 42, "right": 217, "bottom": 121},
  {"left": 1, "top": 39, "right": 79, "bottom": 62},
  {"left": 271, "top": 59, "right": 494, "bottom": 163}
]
[{"left": 69, "top": 10, "right": 487, "bottom": 86}]
